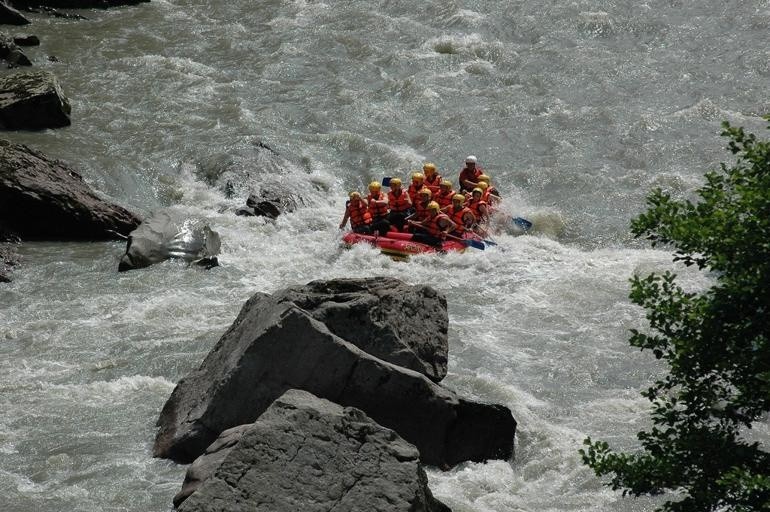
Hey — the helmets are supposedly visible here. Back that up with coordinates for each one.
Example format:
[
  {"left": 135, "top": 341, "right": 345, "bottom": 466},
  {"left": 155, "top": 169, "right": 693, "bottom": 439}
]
[
  {"left": 412, "top": 173, "right": 423, "bottom": 181},
  {"left": 439, "top": 180, "right": 453, "bottom": 190},
  {"left": 453, "top": 194, "right": 465, "bottom": 206},
  {"left": 472, "top": 174, "right": 489, "bottom": 198},
  {"left": 350, "top": 191, "right": 360, "bottom": 199},
  {"left": 427, "top": 201, "right": 440, "bottom": 213},
  {"left": 465, "top": 155, "right": 477, "bottom": 164},
  {"left": 389, "top": 178, "right": 402, "bottom": 186},
  {"left": 420, "top": 188, "right": 431, "bottom": 199},
  {"left": 423, "top": 163, "right": 436, "bottom": 170},
  {"left": 368, "top": 182, "right": 381, "bottom": 191}
]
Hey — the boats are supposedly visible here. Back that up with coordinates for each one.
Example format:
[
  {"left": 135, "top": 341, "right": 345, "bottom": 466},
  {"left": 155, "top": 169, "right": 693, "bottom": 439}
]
[{"left": 338, "top": 206, "right": 508, "bottom": 264}]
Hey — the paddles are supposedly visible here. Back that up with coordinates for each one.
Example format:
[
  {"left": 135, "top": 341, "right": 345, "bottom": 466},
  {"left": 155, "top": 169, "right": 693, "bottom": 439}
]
[
  {"left": 382, "top": 176, "right": 411, "bottom": 186},
  {"left": 407, "top": 220, "right": 498, "bottom": 250},
  {"left": 491, "top": 206, "right": 532, "bottom": 231}
]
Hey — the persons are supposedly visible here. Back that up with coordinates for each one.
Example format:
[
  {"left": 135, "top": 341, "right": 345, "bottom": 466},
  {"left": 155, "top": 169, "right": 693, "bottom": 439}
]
[
  {"left": 459, "top": 154, "right": 484, "bottom": 193},
  {"left": 338, "top": 162, "right": 504, "bottom": 246}
]
